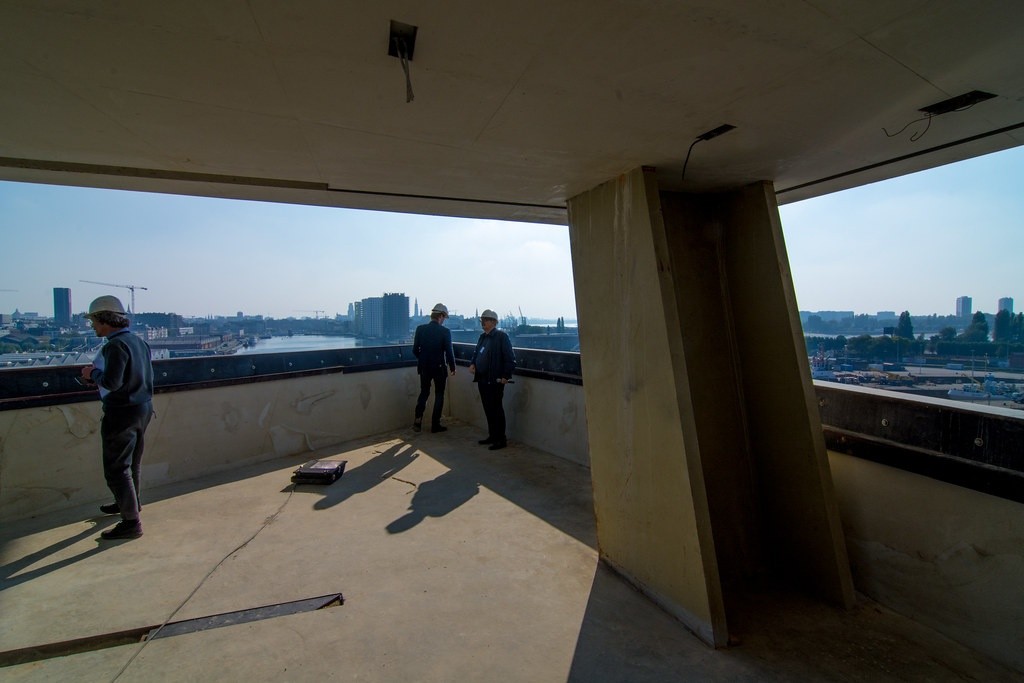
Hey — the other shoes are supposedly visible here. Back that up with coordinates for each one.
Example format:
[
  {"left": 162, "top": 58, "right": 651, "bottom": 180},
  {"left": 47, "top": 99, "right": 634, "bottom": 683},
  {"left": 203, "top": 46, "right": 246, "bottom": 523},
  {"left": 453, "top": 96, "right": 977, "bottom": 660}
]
[
  {"left": 488, "top": 440, "right": 507, "bottom": 450},
  {"left": 412, "top": 423, "right": 421, "bottom": 432},
  {"left": 431, "top": 425, "right": 447, "bottom": 433},
  {"left": 478, "top": 437, "right": 494, "bottom": 444}
]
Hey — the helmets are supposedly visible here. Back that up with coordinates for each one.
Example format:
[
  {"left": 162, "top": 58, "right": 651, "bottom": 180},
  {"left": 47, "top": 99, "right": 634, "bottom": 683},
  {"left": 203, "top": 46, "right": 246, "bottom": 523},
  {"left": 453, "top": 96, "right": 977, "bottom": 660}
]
[
  {"left": 83, "top": 295, "right": 129, "bottom": 319},
  {"left": 478, "top": 309, "right": 499, "bottom": 326},
  {"left": 431, "top": 304, "right": 449, "bottom": 319}
]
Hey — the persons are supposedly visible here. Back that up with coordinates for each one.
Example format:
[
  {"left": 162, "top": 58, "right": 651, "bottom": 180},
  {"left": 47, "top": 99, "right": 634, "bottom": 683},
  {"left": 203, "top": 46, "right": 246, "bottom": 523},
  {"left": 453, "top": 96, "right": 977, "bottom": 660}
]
[
  {"left": 81, "top": 295, "right": 154, "bottom": 540},
  {"left": 469, "top": 310, "right": 516, "bottom": 450},
  {"left": 412, "top": 304, "right": 456, "bottom": 433}
]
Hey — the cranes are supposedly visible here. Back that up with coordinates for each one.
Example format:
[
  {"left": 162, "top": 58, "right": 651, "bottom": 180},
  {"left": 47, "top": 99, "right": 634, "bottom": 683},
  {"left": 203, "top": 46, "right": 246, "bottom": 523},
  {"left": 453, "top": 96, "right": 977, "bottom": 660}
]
[{"left": 78, "top": 279, "right": 149, "bottom": 324}]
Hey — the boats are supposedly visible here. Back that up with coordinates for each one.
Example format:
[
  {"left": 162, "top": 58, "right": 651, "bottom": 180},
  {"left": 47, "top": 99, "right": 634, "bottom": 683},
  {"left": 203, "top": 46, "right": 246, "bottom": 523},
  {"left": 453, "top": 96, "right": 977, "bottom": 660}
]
[{"left": 947, "top": 350, "right": 1024, "bottom": 406}]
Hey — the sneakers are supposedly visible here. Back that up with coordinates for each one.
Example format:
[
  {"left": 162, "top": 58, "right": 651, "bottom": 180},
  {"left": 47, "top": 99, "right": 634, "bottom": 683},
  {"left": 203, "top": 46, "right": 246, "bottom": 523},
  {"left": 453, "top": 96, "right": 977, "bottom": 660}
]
[
  {"left": 101, "top": 520, "right": 143, "bottom": 539},
  {"left": 100, "top": 497, "right": 141, "bottom": 514}
]
